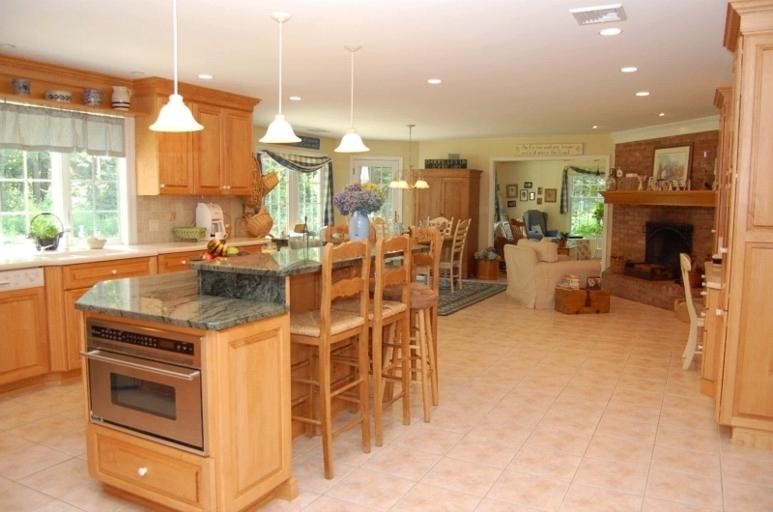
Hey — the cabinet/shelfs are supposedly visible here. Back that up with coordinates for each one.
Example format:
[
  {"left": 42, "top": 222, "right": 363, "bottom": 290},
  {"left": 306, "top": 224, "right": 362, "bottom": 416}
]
[
  {"left": 135, "top": 76, "right": 263, "bottom": 197},
  {"left": 0, "top": 245, "right": 209, "bottom": 398},
  {"left": 411, "top": 168, "right": 484, "bottom": 278}
]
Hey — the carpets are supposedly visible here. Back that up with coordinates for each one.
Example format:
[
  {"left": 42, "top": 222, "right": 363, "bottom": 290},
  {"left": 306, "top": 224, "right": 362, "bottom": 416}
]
[{"left": 414, "top": 280, "right": 508, "bottom": 316}]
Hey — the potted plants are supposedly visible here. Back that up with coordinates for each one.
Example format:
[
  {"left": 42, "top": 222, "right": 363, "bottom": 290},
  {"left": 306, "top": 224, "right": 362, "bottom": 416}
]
[
  {"left": 28, "top": 213, "right": 65, "bottom": 250},
  {"left": 557, "top": 231, "right": 568, "bottom": 247}
]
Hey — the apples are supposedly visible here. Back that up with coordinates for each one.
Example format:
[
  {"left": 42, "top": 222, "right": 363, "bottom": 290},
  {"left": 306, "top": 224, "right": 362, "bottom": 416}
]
[{"left": 227, "top": 247, "right": 240, "bottom": 255}]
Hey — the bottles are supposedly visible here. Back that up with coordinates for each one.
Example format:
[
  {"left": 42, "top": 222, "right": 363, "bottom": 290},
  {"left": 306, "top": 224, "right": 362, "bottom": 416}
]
[{"left": 604, "top": 165, "right": 624, "bottom": 192}]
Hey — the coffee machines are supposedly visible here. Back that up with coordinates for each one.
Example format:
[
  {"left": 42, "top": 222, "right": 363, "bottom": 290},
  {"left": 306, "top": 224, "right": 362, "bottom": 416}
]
[{"left": 195, "top": 202, "right": 228, "bottom": 241}]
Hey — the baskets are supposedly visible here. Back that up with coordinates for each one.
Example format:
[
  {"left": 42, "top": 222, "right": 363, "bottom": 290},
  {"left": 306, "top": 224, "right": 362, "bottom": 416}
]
[
  {"left": 247, "top": 212, "right": 272, "bottom": 239},
  {"left": 611, "top": 256, "right": 627, "bottom": 274},
  {"left": 262, "top": 172, "right": 279, "bottom": 195},
  {"left": 175, "top": 227, "right": 205, "bottom": 240}
]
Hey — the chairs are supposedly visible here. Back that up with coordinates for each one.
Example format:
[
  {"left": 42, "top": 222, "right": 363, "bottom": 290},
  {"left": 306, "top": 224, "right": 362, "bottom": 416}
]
[
  {"left": 522, "top": 209, "right": 558, "bottom": 240},
  {"left": 679, "top": 253, "right": 705, "bottom": 371},
  {"left": 417, "top": 215, "right": 472, "bottom": 292},
  {"left": 382, "top": 227, "right": 440, "bottom": 424},
  {"left": 331, "top": 234, "right": 411, "bottom": 448},
  {"left": 290, "top": 239, "right": 371, "bottom": 481},
  {"left": 504, "top": 239, "right": 601, "bottom": 310}
]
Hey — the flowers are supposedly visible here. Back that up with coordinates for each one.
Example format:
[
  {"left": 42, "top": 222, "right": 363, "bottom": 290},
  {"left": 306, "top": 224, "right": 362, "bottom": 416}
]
[{"left": 333, "top": 184, "right": 390, "bottom": 216}]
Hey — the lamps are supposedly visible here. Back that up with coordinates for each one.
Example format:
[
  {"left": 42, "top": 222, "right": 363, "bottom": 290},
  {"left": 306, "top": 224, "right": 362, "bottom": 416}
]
[
  {"left": 334, "top": 43, "right": 371, "bottom": 152},
  {"left": 259, "top": 11, "right": 302, "bottom": 144},
  {"left": 388, "top": 124, "right": 429, "bottom": 190},
  {"left": 147, "top": 0, "right": 204, "bottom": 132}
]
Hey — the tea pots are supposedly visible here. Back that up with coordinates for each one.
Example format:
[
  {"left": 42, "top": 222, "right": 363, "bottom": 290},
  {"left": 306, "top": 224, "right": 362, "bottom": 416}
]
[{"left": 111, "top": 85, "right": 133, "bottom": 112}]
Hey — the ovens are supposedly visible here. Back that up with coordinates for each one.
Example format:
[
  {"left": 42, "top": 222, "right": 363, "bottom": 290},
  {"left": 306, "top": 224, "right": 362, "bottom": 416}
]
[{"left": 83, "top": 319, "right": 208, "bottom": 453}]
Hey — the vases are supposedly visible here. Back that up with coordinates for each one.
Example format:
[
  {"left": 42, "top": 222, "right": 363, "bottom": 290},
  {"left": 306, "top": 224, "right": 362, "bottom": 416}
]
[{"left": 348, "top": 210, "right": 370, "bottom": 243}]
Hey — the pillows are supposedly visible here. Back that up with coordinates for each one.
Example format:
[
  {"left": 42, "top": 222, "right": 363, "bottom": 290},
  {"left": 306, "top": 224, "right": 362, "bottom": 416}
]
[{"left": 531, "top": 224, "right": 543, "bottom": 235}]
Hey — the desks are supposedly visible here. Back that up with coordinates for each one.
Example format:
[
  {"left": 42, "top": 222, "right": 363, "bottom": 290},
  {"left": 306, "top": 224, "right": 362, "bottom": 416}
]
[
  {"left": 700, "top": 262, "right": 722, "bottom": 398},
  {"left": 558, "top": 246, "right": 578, "bottom": 261}
]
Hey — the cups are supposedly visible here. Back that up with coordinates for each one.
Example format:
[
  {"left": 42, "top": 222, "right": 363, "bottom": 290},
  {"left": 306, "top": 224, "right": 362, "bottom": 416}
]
[{"left": 10, "top": 78, "right": 30, "bottom": 96}]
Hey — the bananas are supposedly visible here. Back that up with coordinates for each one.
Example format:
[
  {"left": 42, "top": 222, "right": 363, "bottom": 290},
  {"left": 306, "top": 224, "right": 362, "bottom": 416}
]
[{"left": 207, "top": 239, "right": 226, "bottom": 257}]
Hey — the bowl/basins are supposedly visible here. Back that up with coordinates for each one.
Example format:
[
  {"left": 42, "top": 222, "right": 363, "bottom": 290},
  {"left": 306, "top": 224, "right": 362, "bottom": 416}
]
[
  {"left": 45, "top": 91, "right": 71, "bottom": 102},
  {"left": 86, "top": 239, "right": 106, "bottom": 249}
]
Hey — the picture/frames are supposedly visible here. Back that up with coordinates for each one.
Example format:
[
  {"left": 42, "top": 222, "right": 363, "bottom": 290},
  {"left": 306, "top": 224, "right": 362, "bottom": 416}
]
[
  {"left": 506, "top": 181, "right": 557, "bottom": 207},
  {"left": 650, "top": 142, "right": 693, "bottom": 190}
]
